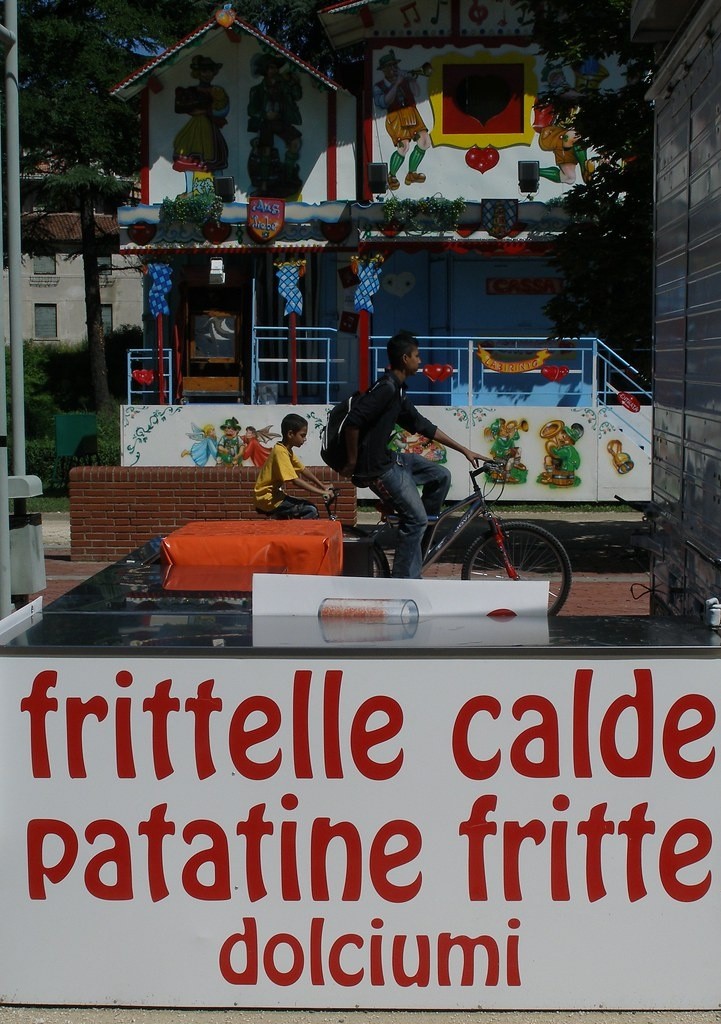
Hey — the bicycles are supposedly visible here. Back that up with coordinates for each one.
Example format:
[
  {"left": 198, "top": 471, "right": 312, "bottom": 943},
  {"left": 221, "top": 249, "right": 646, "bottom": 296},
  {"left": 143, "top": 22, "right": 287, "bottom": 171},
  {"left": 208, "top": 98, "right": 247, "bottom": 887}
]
[
  {"left": 340, "top": 461, "right": 572, "bottom": 620},
  {"left": 255, "top": 482, "right": 390, "bottom": 578}
]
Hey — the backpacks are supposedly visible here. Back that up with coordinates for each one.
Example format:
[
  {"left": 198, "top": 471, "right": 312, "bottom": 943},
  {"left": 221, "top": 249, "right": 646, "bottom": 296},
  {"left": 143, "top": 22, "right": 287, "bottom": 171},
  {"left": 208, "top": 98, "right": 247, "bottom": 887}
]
[{"left": 320, "top": 379, "right": 399, "bottom": 478}]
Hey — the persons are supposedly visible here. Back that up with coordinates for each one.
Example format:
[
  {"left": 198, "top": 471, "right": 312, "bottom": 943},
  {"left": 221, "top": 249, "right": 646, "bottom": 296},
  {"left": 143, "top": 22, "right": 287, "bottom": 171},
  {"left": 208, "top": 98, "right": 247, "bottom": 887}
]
[
  {"left": 353, "top": 342, "right": 493, "bottom": 579},
  {"left": 253, "top": 413, "right": 334, "bottom": 519}
]
